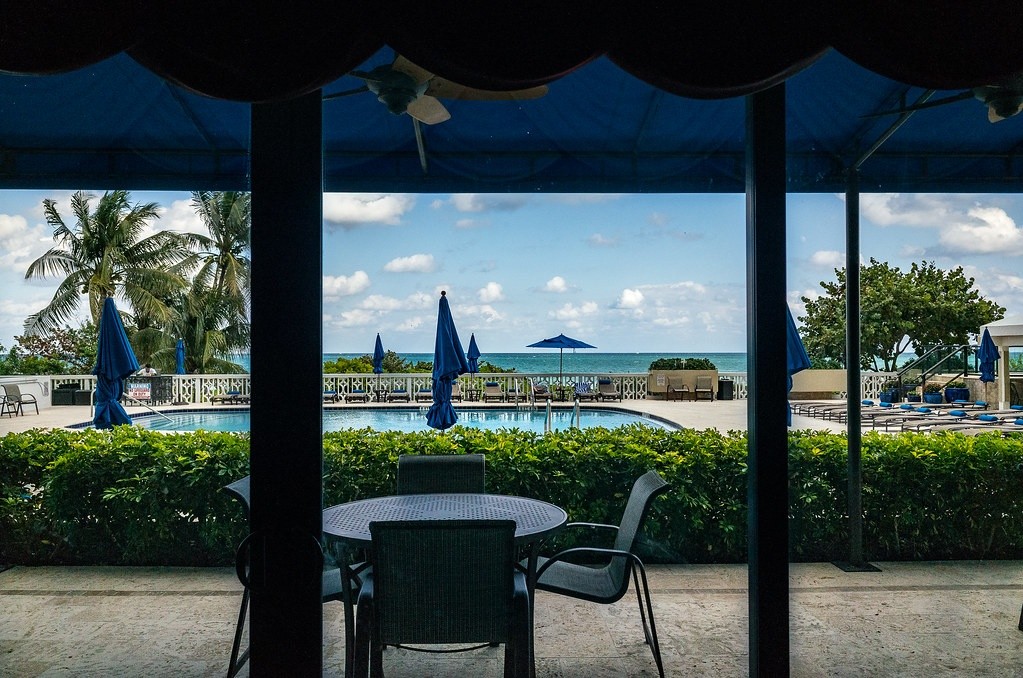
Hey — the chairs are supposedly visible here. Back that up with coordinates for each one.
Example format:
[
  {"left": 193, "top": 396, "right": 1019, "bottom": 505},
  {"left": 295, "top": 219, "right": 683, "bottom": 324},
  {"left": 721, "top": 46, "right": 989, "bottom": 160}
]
[
  {"left": 397, "top": 451, "right": 487, "bottom": 494},
  {"left": 694, "top": 375, "right": 714, "bottom": 403},
  {"left": 235, "top": 393, "right": 251, "bottom": 404},
  {"left": 451, "top": 380, "right": 461, "bottom": 403},
  {"left": 666, "top": 376, "right": 692, "bottom": 402},
  {"left": 414, "top": 387, "right": 433, "bottom": 403},
  {"left": 572, "top": 381, "right": 599, "bottom": 402},
  {"left": 323, "top": 390, "right": 339, "bottom": 404},
  {"left": 533, "top": 467, "right": 675, "bottom": 678},
  {"left": 351, "top": 519, "right": 531, "bottom": 678},
  {"left": 507, "top": 388, "right": 527, "bottom": 403},
  {"left": 533, "top": 380, "right": 554, "bottom": 402},
  {"left": 1, "top": 383, "right": 40, "bottom": 416},
  {"left": 482, "top": 381, "right": 504, "bottom": 403},
  {"left": 214, "top": 475, "right": 372, "bottom": 678},
  {"left": 0, "top": 393, "right": 17, "bottom": 418},
  {"left": 210, "top": 390, "right": 240, "bottom": 405},
  {"left": 790, "top": 397, "right": 1023, "bottom": 440},
  {"left": 596, "top": 378, "right": 622, "bottom": 402},
  {"left": 389, "top": 388, "right": 410, "bottom": 403},
  {"left": 344, "top": 389, "right": 369, "bottom": 404}
]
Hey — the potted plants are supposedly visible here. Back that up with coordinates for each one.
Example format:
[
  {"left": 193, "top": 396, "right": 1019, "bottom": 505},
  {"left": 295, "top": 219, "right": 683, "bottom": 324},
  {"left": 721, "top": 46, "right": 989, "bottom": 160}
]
[
  {"left": 903, "top": 377, "right": 919, "bottom": 395},
  {"left": 924, "top": 382, "right": 943, "bottom": 403},
  {"left": 906, "top": 391, "right": 921, "bottom": 402},
  {"left": 879, "top": 380, "right": 902, "bottom": 403},
  {"left": 944, "top": 380, "right": 971, "bottom": 403}
]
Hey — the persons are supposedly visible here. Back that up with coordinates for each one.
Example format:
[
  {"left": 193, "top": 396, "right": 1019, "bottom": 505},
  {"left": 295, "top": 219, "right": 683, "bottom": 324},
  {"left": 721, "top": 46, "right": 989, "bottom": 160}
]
[{"left": 138, "top": 364, "right": 157, "bottom": 375}]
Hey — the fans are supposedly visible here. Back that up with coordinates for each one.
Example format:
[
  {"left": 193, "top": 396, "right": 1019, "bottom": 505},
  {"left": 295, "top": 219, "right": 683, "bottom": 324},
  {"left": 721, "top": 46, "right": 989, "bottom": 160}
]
[
  {"left": 319, "top": 51, "right": 552, "bottom": 123},
  {"left": 852, "top": 58, "right": 1023, "bottom": 126}
]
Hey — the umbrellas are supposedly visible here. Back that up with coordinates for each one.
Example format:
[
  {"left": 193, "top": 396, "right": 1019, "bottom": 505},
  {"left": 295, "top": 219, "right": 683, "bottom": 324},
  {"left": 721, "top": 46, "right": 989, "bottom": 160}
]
[
  {"left": 527, "top": 332, "right": 598, "bottom": 389},
  {"left": 175, "top": 339, "right": 186, "bottom": 375},
  {"left": 784, "top": 300, "right": 812, "bottom": 427},
  {"left": 978, "top": 327, "right": 1001, "bottom": 403},
  {"left": 373, "top": 334, "right": 386, "bottom": 400},
  {"left": 467, "top": 333, "right": 481, "bottom": 395},
  {"left": 425, "top": 291, "right": 469, "bottom": 432},
  {"left": 91, "top": 296, "right": 139, "bottom": 429}
]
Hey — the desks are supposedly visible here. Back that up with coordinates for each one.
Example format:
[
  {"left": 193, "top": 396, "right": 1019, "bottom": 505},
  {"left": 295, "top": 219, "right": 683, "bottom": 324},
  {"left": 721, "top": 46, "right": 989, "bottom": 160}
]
[
  {"left": 466, "top": 389, "right": 482, "bottom": 402},
  {"left": 554, "top": 388, "right": 571, "bottom": 402},
  {"left": 372, "top": 389, "right": 389, "bottom": 403},
  {"left": 322, "top": 493, "right": 569, "bottom": 678}
]
